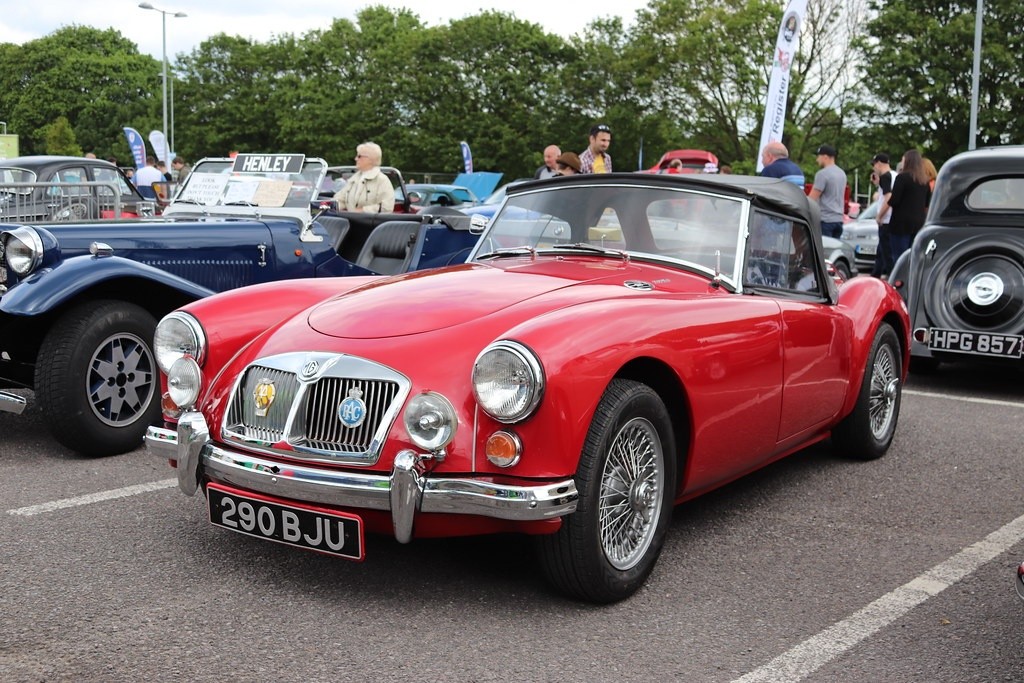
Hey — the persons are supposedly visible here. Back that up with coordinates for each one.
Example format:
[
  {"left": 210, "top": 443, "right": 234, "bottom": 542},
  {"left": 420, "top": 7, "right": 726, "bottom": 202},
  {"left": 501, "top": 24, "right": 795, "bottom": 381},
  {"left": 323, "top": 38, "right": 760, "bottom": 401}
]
[
  {"left": 534, "top": 124, "right": 613, "bottom": 179},
  {"left": 333, "top": 142, "right": 395, "bottom": 214},
  {"left": 77, "top": 152, "right": 192, "bottom": 206},
  {"left": 759, "top": 142, "right": 805, "bottom": 191},
  {"left": 720, "top": 166, "right": 731, "bottom": 175},
  {"left": 859, "top": 149, "right": 937, "bottom": 283},
  {"left": 669, "top": 159, "right": 682, "bottom": 173},
  {"left": 807, "top": 145, "right": 847, "bottom": 241}
]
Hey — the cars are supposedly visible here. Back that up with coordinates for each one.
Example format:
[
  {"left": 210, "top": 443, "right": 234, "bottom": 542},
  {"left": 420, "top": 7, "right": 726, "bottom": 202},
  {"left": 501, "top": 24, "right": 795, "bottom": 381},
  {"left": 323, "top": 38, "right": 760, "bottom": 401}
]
[
  {"left": 393, "top": 172, "right": 505, "bottom": 207},
  {"left": 888, "top": 144, "right": 1024, "bottom": 367},
  {"left": 840, "top": 192, "right": 891, "bottom": 269},
  {"left": 0, "top": 157, "right": 164, "bottom": 221},
  {"left": 459, "top": 178, "right": 546, "bottom": 219},
  {"left": 139, "top": 171, "right": 908, "bottom": 605},
  {"left": 0, "top": 155, "right": 491, "bottom": 461}
]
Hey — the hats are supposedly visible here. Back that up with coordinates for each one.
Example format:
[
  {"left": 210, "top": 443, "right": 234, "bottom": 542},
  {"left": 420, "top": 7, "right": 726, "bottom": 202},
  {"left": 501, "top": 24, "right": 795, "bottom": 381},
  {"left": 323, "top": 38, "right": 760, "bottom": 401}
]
[
  {"left": 870, "top": 153, "right": 889, "bottom": 163},
  {"left": 590, "top": 124, "right": 611, "bottom": 135},
  {"left": 812, "top": 145, "right": 836, "bottom": 155},
  {"left": 556, "top": 152, "right": 583, "bottom": 173}
]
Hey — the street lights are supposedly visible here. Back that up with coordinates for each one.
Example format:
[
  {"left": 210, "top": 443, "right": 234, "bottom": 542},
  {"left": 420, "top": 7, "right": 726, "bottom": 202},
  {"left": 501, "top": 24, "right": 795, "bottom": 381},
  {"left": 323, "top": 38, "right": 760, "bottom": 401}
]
[{"left": 139, "top": 2, "right": 188, "bottom": 170}]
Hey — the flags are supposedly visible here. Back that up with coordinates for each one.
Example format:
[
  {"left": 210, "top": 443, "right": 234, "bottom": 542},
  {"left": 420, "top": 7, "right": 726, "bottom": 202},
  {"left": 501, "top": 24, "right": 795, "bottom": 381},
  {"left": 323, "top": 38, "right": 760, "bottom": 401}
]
[{"left": 756, "top": 0, "right": 807, "bottom": 173}]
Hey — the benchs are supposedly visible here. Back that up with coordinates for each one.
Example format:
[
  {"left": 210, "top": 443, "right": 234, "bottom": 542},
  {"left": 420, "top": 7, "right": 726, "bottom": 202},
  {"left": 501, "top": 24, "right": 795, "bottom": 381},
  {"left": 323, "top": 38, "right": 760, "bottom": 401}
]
[{"left": 656, "top": 253, "right": 816, "bottom": 292}]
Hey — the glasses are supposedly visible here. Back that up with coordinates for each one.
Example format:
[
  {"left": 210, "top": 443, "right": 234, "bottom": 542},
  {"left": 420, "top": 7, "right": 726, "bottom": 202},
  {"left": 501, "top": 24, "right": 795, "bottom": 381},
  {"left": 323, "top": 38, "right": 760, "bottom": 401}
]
[
  {"left": 871, "top": 161, "right": 878, "bottom": 167},
  {"left": 358, "top": 154, "right": 368, "bottom": 158}
]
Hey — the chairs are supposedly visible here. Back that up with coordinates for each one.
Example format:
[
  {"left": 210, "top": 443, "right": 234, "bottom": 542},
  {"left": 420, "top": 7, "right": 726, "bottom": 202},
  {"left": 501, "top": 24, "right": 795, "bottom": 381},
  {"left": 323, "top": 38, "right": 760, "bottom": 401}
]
[
  {"left": 356, "top": 221, "right": 421, "bottom": 276},
  {"left": 314, "top": 216, "right": 350, "bottom": 252}
]
